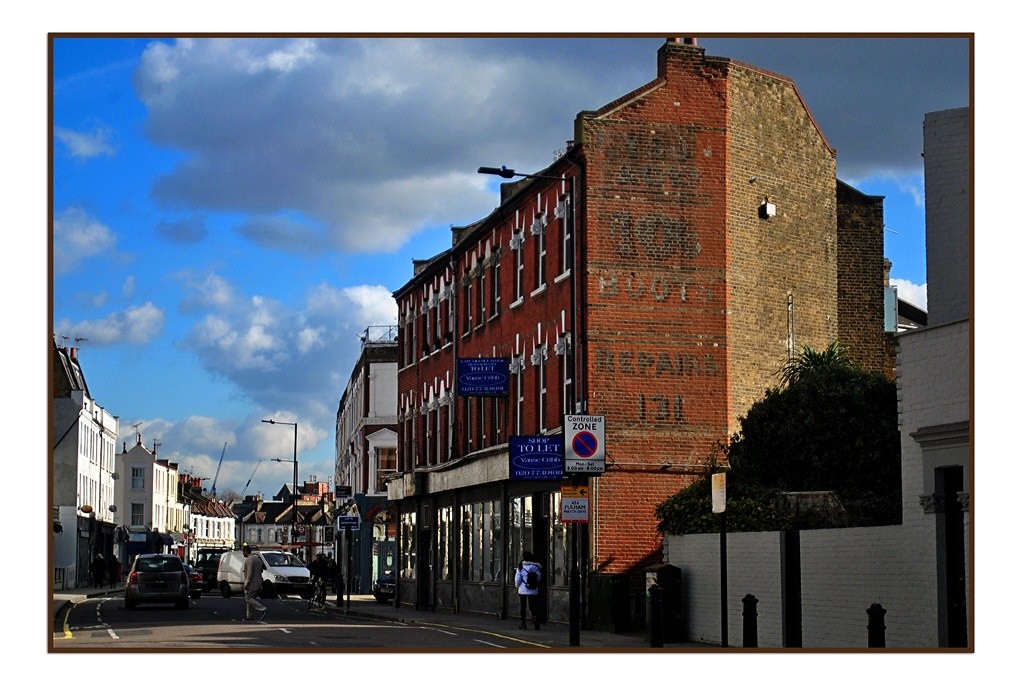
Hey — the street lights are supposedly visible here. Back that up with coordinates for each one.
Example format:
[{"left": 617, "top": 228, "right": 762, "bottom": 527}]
[
  {"left": 710, "top": 467, "right": 728, "bottom": 648},
  {"left": 271, "top": 459, "right": 298, "bottom": 523},
  {"left": 261, "top": 419, "right": 297, "bottom": 524}
]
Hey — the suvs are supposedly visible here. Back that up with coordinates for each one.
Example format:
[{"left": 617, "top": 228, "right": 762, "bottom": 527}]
[{"left": 125, "top": 553, "right": 189, "bottom": 611}]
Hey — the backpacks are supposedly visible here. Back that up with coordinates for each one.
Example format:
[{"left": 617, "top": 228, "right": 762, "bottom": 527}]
[{"left": 520, "top": 567, "right": 538, "bottom": 589}]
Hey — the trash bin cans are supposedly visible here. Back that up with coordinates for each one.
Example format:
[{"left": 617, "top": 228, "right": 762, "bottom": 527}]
[{"left": 642, "top": 563, "right": 683, "bottom": 643}]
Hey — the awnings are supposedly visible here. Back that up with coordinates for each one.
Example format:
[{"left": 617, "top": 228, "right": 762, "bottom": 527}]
[{"left": 157, "top": 535, "right": 174, "bottom": 545}]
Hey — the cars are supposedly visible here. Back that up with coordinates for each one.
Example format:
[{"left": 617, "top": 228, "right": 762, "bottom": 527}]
[
  {"left": 183, "top": 563, "right": 202, "bottom": 600},
  {"left": 373, "top": 571, "right": 396, "bottom": 601}
]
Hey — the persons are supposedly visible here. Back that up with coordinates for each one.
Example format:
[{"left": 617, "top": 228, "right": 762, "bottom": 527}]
[
  {"left": 243, "top": 546, "right": 268, "bottom": 620},
  {"left": 515, "top": 552, "right": 541, "bottom": 630},
  {"left": 89, "top": 554, "right": 118, "bottom": 589},
  {"left": 309, "top": 554, "right": 327, "bottom": 606}
]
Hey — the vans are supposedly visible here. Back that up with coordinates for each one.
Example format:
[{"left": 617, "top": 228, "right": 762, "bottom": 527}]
[{"left": 217, "top": 551, "right": 310, "bottom": 600}]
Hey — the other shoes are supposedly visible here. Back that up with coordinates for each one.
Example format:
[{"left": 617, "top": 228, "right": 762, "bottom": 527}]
[
  {"left": 260, "top": 609, "right": 266, "bottom": 620},
  {"left": 535, "top": 625, "right": 540, "bottom": 631},
  {"left": 243, "top": 616, "right": 253, "bottom": 620},
  {"left": 518, "top": 626, "right": 527, "bottom": 630}
]
